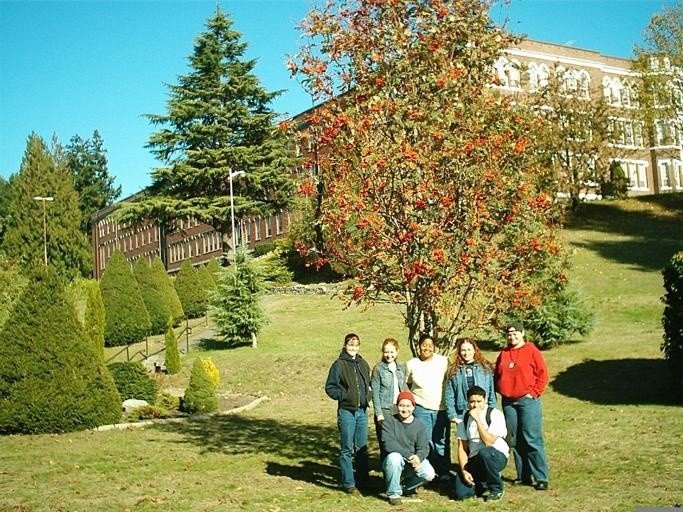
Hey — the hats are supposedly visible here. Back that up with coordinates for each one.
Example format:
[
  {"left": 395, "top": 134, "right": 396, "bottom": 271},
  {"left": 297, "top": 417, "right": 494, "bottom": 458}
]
[
  {"left": 396, "top": 391, "right": 415, "bottom": 405},
  {"left": 506, "top": 322, "right": 525, "bottom": 333}
]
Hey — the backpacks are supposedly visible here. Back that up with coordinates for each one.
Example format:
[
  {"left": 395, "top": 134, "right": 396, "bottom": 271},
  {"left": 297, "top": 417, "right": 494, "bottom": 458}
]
[{"left": 486, "top": 408, "right": 514, "bottom": 448}]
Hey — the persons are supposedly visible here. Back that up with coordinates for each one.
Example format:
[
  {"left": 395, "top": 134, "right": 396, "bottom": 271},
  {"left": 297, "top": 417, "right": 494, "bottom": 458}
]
[
  {"left": 404, "top": 335, "right": 449, "bottom": 482},
  {"left": 444, "top": 337, "right": 499, "bottom": 424},
  {"left": 324, "top": 333, "right": 372, "bottom": 493},
  {"left": 454, "top": 386, "right": 510, "bottom": 502},
  {"left": 494, "top": 322, "right": 549, "bottom": 490},
  {"left": 370, "top": 338, "right": 409, "bottom": 446},
  {"left": 380, "top": 391, "right": 436, "bottom": 505}
]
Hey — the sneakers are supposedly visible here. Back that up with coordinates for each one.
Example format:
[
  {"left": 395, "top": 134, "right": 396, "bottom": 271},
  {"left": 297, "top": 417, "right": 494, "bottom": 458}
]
[
  {"left": 388, "top": 495, "right": 404, "bottom": 507},
  {"left": 342, "top": 485, "right": 359, "bottom": 493},
  {"left": 485, "top": 487, "right": 506, "bottom": 503},
  {"left": 511, "top": 478, "right": 534, "bottom": 487},
  {"left": 535, "top": 480, "right": 549, "bottom": 490}
]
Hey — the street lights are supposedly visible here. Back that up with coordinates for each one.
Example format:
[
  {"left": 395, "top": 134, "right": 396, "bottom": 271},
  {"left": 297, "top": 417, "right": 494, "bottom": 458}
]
[
  {"left": 228, "top": 167, "right": 246, "bottom": 271},
  {"left": 33, "top": 195, "right": 56, "bottom": 266}
]
